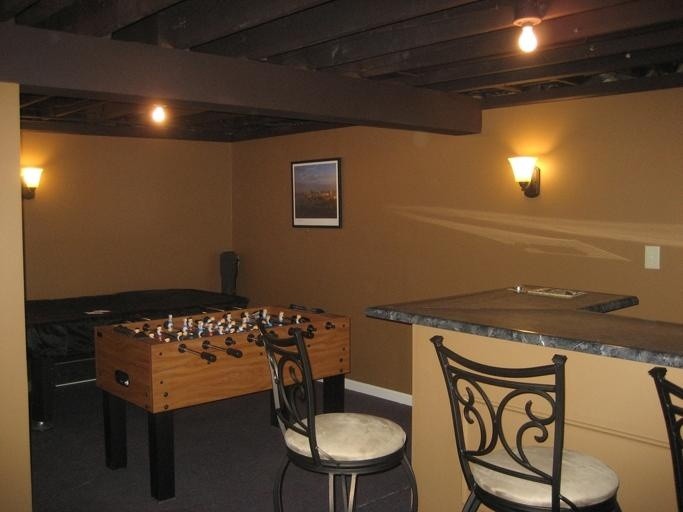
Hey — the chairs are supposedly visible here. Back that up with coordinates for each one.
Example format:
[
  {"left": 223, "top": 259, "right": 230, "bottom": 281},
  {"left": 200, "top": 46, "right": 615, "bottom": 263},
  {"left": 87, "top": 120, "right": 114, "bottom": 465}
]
[
  {"left": 429, "top": 335, "right": 623, "bottom": 512},
  {"left": 647, "top": 366, "right": 683, "bottom": 512},
  {"left": 255, "top": 321, "right": 418, "bottom": 512}
]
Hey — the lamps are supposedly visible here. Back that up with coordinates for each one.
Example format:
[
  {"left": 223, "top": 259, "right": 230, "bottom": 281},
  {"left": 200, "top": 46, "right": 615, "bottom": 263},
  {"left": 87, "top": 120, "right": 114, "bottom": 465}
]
[
  {"left": 152, "top": 104, "right": 167, "bottom": 124},
  {"left": 507, "top": 157, "right": 540, "bottom": 198},
  {"left": 19, "top": 167, "right": 44, "bottom": 199},
  {"left": 512, "top": 0, "right": 543, "bottom": 55}
]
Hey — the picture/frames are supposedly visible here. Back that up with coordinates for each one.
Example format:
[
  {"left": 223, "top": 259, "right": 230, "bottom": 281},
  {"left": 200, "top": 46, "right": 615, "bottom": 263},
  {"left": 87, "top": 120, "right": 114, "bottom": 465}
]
[{"left": 289, "top": 157, "right": 343, "bottom": 229}]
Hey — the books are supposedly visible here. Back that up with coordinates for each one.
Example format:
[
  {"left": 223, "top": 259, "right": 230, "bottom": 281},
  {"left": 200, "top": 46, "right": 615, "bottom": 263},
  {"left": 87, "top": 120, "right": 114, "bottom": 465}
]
[{"left": 526, "top": 288, "right": 586, "bottom": 299}]
